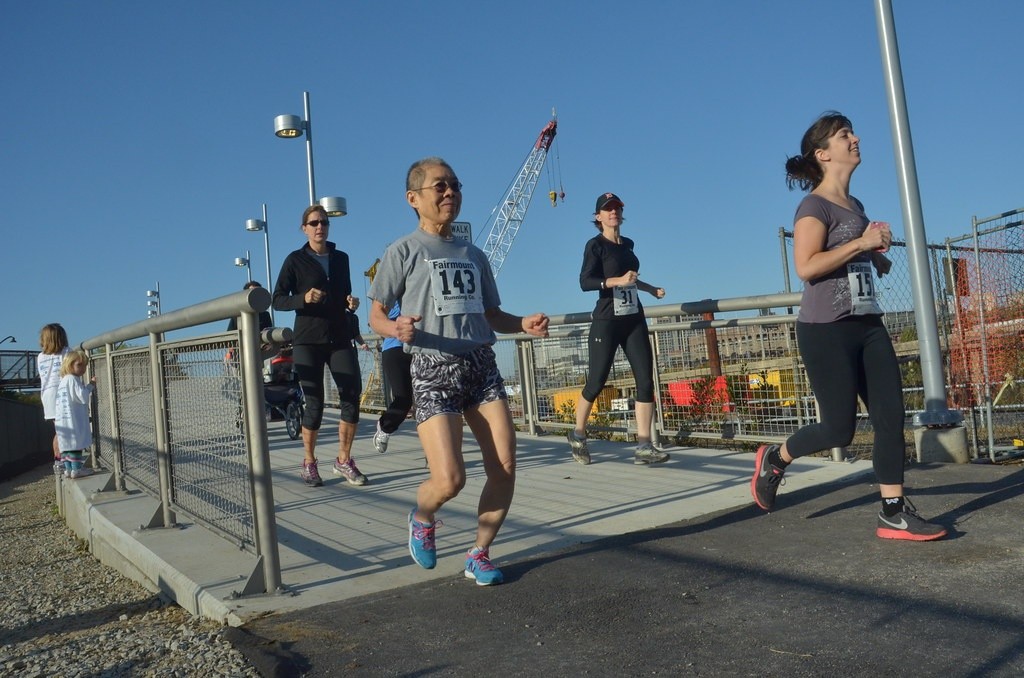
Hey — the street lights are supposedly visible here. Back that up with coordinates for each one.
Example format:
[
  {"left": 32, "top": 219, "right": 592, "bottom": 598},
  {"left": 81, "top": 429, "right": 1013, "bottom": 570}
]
[
  {"left": 146, "top": 289, "right": 165, "bottom": 342},
  {"left": 236, "top": 258, "right": 251, "bottom": 283},
  {"left": 245, "top": 219, "right": 275, "bottom": 327},
  {"left": 273, "top": 115, "right": 348, "bottom": 217}
]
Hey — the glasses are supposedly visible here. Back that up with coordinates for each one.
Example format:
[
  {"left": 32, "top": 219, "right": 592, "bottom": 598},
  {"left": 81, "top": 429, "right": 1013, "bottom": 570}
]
[
  {"left": 303, "top": 220, "right": 329, "bottom": 227},
  {"left": 411, "top": 181, "right": 462, "bottom": 192}
]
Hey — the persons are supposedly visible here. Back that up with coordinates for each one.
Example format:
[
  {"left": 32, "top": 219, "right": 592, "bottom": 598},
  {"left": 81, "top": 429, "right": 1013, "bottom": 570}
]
[
  {"left": 567, "top": 192, "right": 671, "bottom": 465},
  {"left": 753, "top": 109, "right": 949, "bottom": 541},
  {"left": 37, "top": 323, "right": 97, "bottom": 479},
  {"left": 366, "top": 155, "right": 550, "bottom": 586},
  {"left": 227, "top": 204, "right": 369, "bottom": 486}
]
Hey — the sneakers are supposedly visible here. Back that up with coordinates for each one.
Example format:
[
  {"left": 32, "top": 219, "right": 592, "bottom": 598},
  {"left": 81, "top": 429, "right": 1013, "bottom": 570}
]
[
  {"left": 634, "top": 444, "right": 669, "bottom": 465},
  {"left": 876, "top": 505, "right": 948, "bottom": 541},
  {"left": 333, "top": 456, "right": 369, "bottom": 486},
  {"left": 407, "top": 507, "right": 442, "bottom": 569},
  {"left": 463, "top": 544, "right": 504, "bottom": 585},
  {"left": 299, "top": 457, "right": 322, "bottom": 487},
  {"left": 751, "top": 444, "right": 786, "bottom": 510},
  {"left": 567, "top": 428, "right": 589, "bottom": 465},
  {"left": 374, "top": 419, "right": 391, "bottom": 454}
]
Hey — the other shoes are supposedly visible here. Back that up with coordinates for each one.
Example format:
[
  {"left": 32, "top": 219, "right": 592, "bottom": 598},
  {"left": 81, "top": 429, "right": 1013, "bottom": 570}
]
[
  {"left": 63, "top": 470, "right": 72, "bottom": 478},
  {"left": 71, "top": 468, "right": 95, "bottom": 479},
  {"left": 53, "top": 464, "right": 65, "bottom": 475}
]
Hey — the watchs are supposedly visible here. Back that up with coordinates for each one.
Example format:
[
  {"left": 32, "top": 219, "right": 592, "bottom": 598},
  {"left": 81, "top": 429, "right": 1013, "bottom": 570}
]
[{"left": 90, "top": 381, "right": 96, "bottom": 384}]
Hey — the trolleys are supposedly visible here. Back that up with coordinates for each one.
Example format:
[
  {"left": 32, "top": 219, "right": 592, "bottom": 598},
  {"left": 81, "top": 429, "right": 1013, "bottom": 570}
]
[{"left": 237, "top": 328, "right": 307, "bottom": 441}]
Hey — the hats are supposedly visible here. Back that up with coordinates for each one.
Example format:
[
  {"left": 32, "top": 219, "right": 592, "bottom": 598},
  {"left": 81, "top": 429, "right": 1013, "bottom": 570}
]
[{"left": 596, "top": 193, "right": 622, "bottom": 212}]
